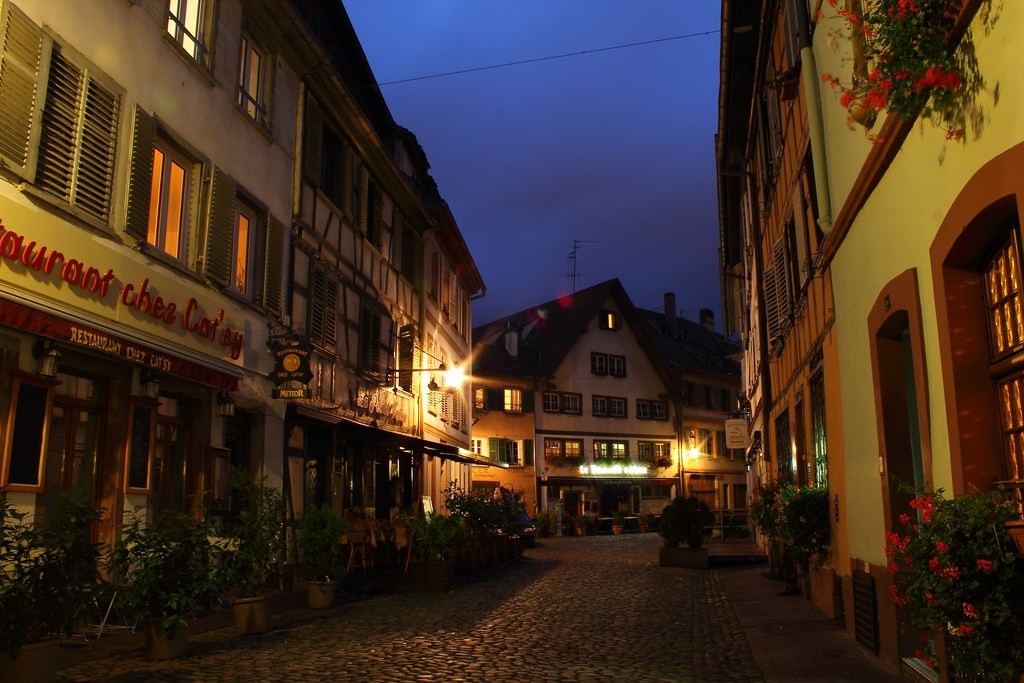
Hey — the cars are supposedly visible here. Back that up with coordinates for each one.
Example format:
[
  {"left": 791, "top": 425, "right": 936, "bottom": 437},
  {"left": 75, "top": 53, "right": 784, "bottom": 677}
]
[{"left": 499, "top": 509, "right": 536, "bottom": 546}]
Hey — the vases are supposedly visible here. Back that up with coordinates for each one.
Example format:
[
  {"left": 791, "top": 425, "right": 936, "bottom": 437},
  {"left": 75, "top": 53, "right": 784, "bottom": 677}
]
[
  {"left": 760, "top": 508, "right": 774, "bottom": 524},
  {"left": 769, "top": 543, "right": 790, "bottom": 574}
]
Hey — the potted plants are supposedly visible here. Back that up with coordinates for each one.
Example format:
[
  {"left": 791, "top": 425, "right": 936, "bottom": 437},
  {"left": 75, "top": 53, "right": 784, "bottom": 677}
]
[
  {"left": 658, "top": 497, "right": 715, "bottom": 569},
  {"left": 612, "top": 515, "right": 624, "bottom": 534},
  {"left": 573, "top": 516, "right": 588, "bottom": 536},
  {"left": 0, "top": 482, "right": 102, "bottom": 683},
  {"left": 638, "top": 515, "right": 648, "bottom": 532},
  {"left": 200, "top": 464, "right": 295, "bottom": 636},
  {"left": 490, "top": 501, "right": 508, "bottom": 563},
  {"left": 403, "top": 506, "right": 465, "bottom": 590},
  {"left": 476, "top": 500, "right": 497, "bottom": 567},
  {"left": 445, "top": 483, "right": 470, "bottom": 583},
  {"left": 110, "top": 505, "right": 220, "bottom": 661},
  {"left": 467, "top": 498, "right": 481, "bottom": 574},
  {"left": 503, "top": 497, "right": 519, "bottom": 558},
  {"left": 289, "top": 503, "right": 344, "bottom": 609}
]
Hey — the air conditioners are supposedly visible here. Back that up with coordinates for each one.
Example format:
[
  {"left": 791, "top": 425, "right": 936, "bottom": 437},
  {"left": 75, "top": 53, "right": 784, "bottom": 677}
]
[{"left": 359, "top": 313, "right": 397, "bottom": 387}]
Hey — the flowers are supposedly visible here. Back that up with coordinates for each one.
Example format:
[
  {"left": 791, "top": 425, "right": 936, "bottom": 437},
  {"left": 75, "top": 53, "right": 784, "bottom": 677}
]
[
  {"left": 812, "top": 0, "right": 966, "bottom": 146},
  {"left": 885, "top": 473, "right": 1024, "bottom": 683},
  {"left": 748, "top": 477, "right": 830, "bottom": 553}
]
